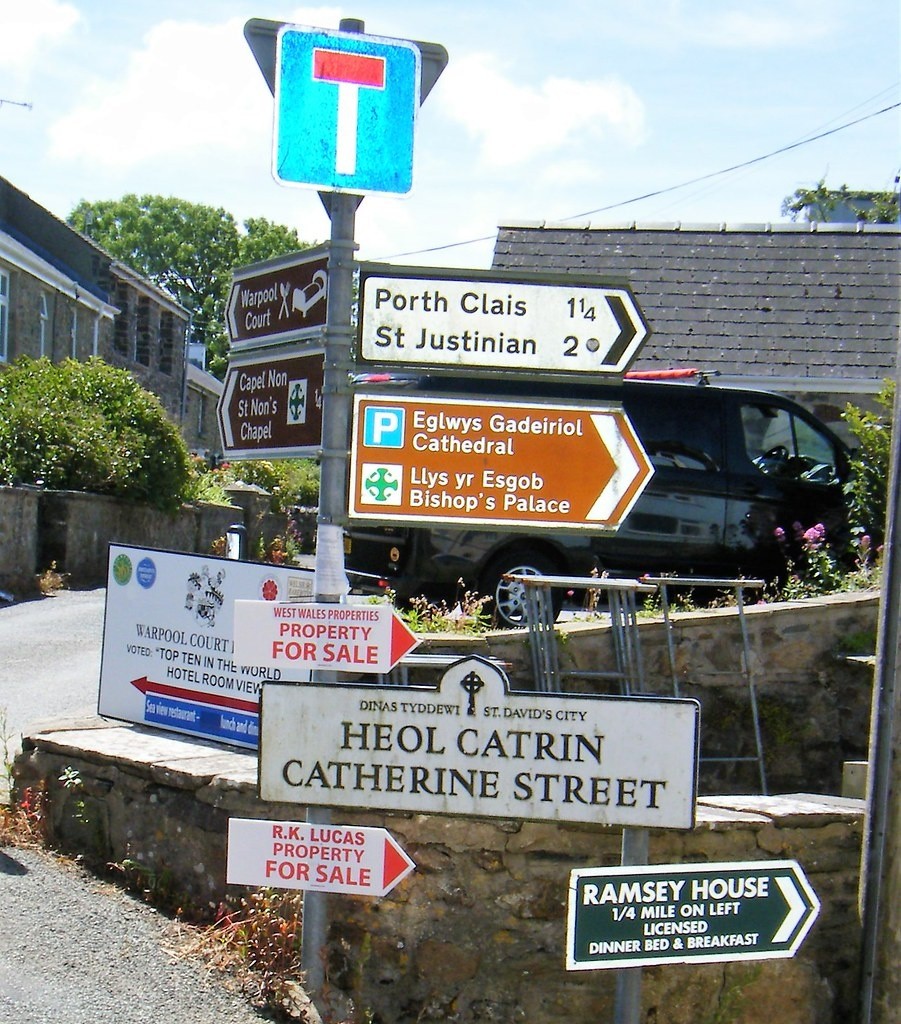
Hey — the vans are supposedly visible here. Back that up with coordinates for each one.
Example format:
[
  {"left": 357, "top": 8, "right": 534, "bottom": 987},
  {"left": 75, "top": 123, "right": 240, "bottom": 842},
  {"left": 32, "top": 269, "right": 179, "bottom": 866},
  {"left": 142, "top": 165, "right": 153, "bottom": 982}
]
[{"left": 342, "top": 363, "right": 885, "bottom": 631}]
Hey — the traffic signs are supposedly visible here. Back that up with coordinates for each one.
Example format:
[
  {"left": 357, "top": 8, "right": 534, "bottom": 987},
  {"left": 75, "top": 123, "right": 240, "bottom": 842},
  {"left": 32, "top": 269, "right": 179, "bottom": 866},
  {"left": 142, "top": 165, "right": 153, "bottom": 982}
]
[
  {"left": 224, "top": 816, "right": 420, "bottom": 903},
  {"left": 565, "top": 858, "right": 821, "bottom": 970},
  {"left": 213, "top": 354, "right": 324, "bottom": 462},
  {"left": 349, "top": 392, "right": 658, "bottom": 539},
  {"left": 353, "top": 265, "right": 649, "bottom": 379},
  {"left": 219, "top": 247, "right": 328, "bottom": 352},
  {"left": 230, "top": 597, "right": 427, "bottom": 678}
]
